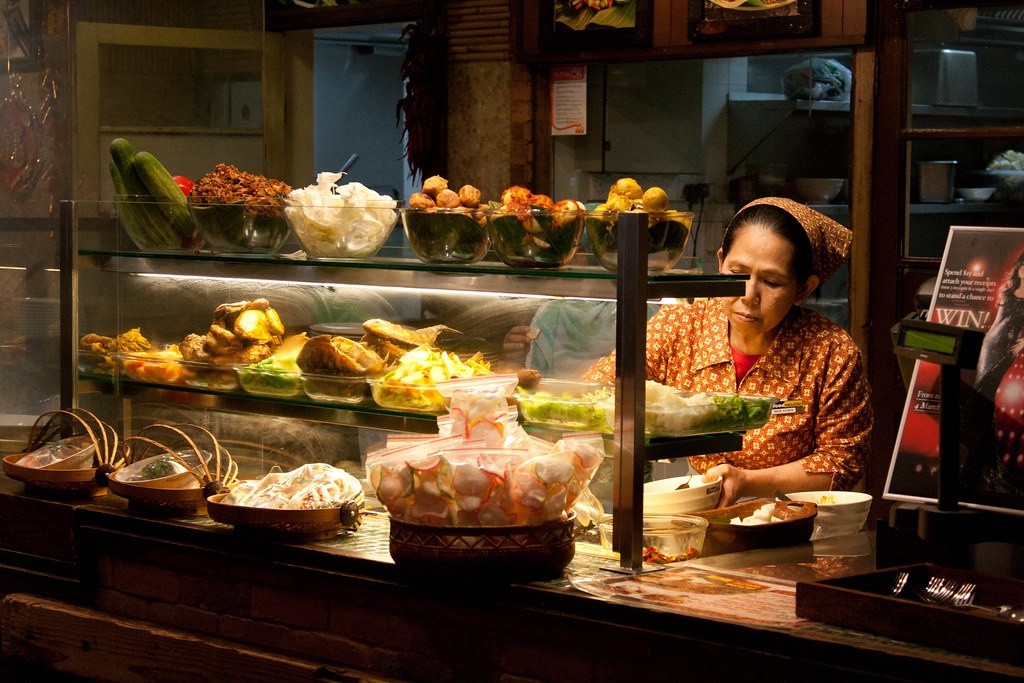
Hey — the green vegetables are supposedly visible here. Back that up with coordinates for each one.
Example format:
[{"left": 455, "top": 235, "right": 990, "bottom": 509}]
[
  {"left": 380, "top": 343, "right": 609, "bottom": 426},
  {"left": 687, "top": 393, "right": 773, "bottom": 431}
]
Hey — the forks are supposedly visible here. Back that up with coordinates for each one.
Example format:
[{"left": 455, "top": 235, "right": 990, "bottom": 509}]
[{"left": 889, "top": 572, "right": 1003, "bottom": 614}]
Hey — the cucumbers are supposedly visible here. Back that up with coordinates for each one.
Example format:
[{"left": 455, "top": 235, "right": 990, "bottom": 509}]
[{"left": 108, "top": 138, "right": 200, "bottom": 250}]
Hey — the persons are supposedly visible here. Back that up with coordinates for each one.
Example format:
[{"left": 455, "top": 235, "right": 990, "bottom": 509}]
[
  {"left": 973, "top": 250, "right": 1024, "bottom": 404},
  {"left": 501, "top": 299, "right": 660, "bottom": 513},
  {"left": 586, "top": 197, "right": 874, "bottom": 511}
]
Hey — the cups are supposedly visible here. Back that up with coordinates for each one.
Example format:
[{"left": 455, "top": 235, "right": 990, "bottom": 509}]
[{"left": 914, "top": 160, "right": 959, "bottom": 202}]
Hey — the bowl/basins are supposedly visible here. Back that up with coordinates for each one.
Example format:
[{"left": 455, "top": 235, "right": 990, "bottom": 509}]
[
  {"left": 584, "top": 213, "right": 694, "bottom": 271},
  {"left": 115, "top": 449, "right": 212, "bottom": 489},
  {"left": 959, "top": 188, "right": 996, "bottom": 203},
  {"left": 795, "top": 178, "right": 845, "bottom": 204},
  {"left": 643, "top": 474, "right": 723, "bottom": 515},
  {"left": 486, "top": 211, "right": 585, "bottom": 268},
  {"left": 115, "top": 195, "right": 205, "bottom": 254},
  {"left": 14, "top": 436, "right": 95, "bottom": 470},
  {"left": 399, "top": 207, "right": 491, "bottom": 264},
  {"left": 599, "top": 512, "right": 709, "bottom": 563},
  {"left": 282, "top": 201, "right": 406, "bottom": 262},
  {"left": 77, "top": 350, "right": 779, "bottom": 437},
  {"left": 186, "top": 196, "right": 291, "bottom": 258},
  {"left": 775, "top": 491, "right": 873, "bottom": 540}
]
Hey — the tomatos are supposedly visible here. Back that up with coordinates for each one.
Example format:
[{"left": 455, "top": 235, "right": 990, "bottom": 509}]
[{"left": 171, "top": 175, "right": 194, "bottom": 197}]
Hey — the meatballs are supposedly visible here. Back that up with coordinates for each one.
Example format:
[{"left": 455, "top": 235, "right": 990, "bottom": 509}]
[{"left": 404, "top": 175, "right": 694, "bottom": 257}]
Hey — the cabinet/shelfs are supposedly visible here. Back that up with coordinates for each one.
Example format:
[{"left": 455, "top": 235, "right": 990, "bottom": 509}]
[
  {"left": 59, "top": 199, "right": 751, "bottom": 572},
  {"left": 729, "top": 97, "right": 1023, "bottom": 215}
]
